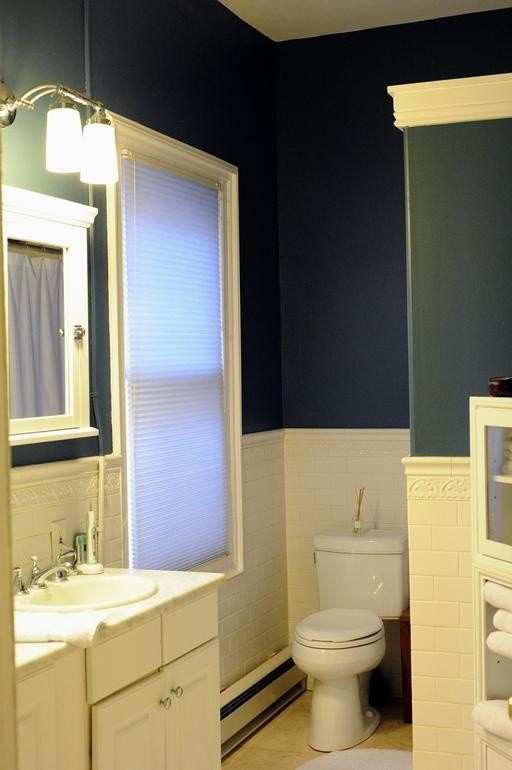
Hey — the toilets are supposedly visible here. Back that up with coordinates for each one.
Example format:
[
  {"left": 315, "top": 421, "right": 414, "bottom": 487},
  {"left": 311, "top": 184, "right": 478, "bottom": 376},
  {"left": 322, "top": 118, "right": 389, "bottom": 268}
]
[{"left": 292, "top": 531, "right": 408, "bottom": 752}]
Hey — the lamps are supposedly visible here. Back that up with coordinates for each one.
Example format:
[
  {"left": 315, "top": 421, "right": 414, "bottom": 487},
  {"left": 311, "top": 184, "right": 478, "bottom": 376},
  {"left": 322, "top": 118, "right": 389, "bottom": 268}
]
[{"left": 0, "top": 77, "right": 120, "bottom": 190}]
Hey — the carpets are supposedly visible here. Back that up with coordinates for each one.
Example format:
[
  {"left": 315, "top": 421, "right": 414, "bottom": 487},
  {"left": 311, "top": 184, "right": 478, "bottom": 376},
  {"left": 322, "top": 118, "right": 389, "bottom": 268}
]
[{"left": 289, "top": 747, "right": 412, "bottom": 770}]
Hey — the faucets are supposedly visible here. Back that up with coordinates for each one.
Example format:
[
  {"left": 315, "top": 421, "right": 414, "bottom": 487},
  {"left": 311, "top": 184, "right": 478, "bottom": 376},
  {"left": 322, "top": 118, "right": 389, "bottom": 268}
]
[{"left": 28, "top": 555, "right": 77, "bottom": 585}]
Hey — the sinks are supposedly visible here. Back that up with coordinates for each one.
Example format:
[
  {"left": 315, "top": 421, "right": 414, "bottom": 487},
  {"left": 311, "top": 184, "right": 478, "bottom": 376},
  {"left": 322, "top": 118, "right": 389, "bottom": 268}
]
[{"left": 15, "top": 573, "right": 159, "bottom": 612}]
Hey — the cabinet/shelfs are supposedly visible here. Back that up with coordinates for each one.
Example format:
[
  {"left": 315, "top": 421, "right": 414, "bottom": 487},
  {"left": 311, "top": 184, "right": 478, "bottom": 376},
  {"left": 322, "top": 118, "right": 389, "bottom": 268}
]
[
  {"left": 13, "top": 590, "right": 223, "bottom": 769},
  {"left": 469, "top": 395, "right": 512, "bottom": 770},
  {"left": 3, "top": 187, "right": 110, "bottom": 447}
]
